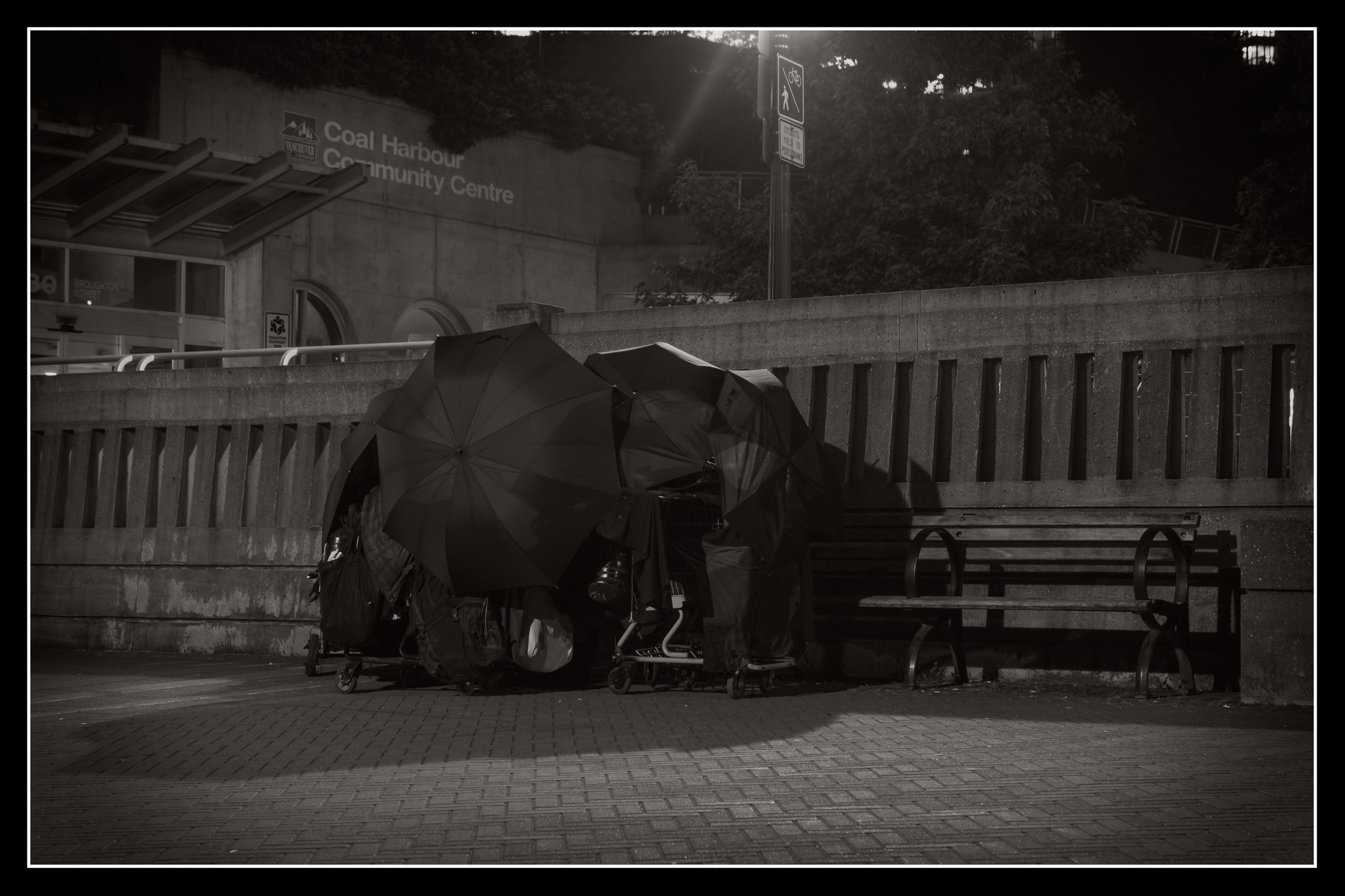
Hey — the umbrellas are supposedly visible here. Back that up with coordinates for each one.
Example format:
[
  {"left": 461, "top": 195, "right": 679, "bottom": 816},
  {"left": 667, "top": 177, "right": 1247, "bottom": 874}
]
[
  {"left": 374, "top": 321, "right": 843, "bottom": 599},
  {"left": 320, "top": 387, "right": 401, "bottom": 557}
]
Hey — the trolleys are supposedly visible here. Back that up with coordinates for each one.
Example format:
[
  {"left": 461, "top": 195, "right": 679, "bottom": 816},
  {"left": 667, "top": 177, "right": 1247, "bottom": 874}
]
[
  {"left": 607, "top": 550, "right": 795, "bottom": 700},
  {"left": 305, "top": 541, "right": 423, "bottom": 694}
]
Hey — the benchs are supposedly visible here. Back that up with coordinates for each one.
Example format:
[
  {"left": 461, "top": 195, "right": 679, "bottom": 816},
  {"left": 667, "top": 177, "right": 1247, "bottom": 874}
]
[{"left": 808, "top": 510, "right": 1203, "bottom": 699}]
